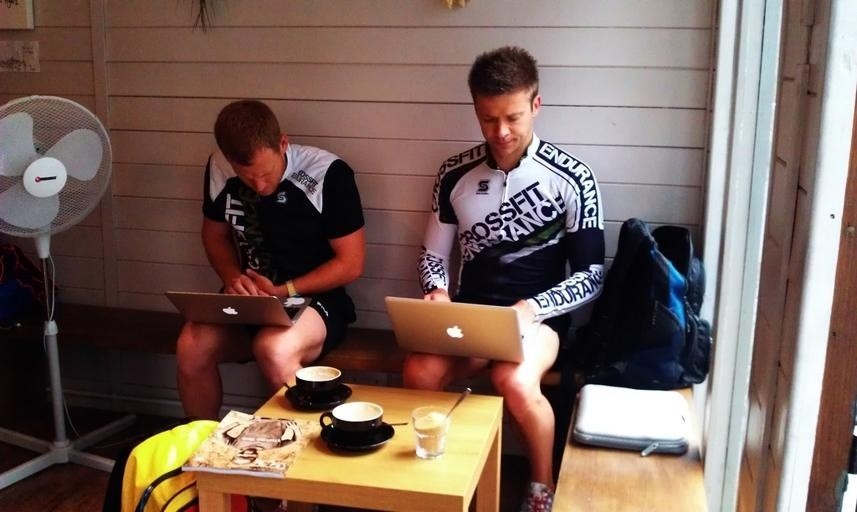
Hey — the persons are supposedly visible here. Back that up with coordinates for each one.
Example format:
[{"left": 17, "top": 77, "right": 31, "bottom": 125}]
[
  {"left": 402, "top": 45, "right": 605, "bottom": 512},
  {"left": 175, "top": 98, "right": 366, "bottom": 420}
]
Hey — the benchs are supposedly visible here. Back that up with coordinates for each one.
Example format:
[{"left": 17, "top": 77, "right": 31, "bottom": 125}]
[{"left": 551, "top": 385, "right": 708, "bottom": 512}]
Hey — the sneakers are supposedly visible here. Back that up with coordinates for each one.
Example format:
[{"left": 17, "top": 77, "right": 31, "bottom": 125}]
[{"left": 519, "top": 482, "right": 554, "bottom": 512}]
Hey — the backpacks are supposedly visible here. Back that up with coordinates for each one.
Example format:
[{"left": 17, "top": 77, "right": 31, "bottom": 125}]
[
  {"left": 101, "top": 415, "right": 256, "bottom": 512},
  {"left": 557, "top": 218, "right": 710, "bottom": 391}
]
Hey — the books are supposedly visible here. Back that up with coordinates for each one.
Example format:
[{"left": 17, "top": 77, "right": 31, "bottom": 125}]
[{"left": 180, "top": 409, "right": 326, "bottom": 481}]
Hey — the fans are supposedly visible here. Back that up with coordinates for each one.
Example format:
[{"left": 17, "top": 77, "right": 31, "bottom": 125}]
[{"left": 0, "top": 95, "right": 137, "bottom": 490}]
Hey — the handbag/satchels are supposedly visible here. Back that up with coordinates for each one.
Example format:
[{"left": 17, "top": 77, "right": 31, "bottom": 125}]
[{"left": 573, "top": 384, "right": 690, "bottom": 457}]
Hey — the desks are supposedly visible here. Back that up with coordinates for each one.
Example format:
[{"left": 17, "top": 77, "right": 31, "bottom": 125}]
[{"left": 197, "top": 376, "right": 504, "bottom": 512}]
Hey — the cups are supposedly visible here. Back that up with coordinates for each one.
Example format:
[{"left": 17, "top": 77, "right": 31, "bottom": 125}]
[
  {"left": 413, "top": 403, "right": 450, "bottom": 460},
  {"left": 318, "top": 401, "right": 385, "bottom": 438},
  {"left": 296, "top": 365, "right": 343, "bottom": 398}
]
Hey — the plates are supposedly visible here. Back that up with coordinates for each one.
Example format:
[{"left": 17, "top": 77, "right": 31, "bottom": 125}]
[
  {"left": 315, "top": 420, "right": 395, "bottom": 453},
  {"left": 281, "top": 382, "right": 352, "bottom": 413}
]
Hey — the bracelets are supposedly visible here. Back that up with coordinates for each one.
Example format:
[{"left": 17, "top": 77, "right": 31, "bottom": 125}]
[{"left": 285, "top": 280, "right": 300, "bottom": 298}]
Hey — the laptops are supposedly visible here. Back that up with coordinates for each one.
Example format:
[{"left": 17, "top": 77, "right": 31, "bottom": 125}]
[
  {"left": 165, "top": 291, "right": 312, "bottom": 326},
  {"left": 385, "top": 295, "right": 536, "bottom": 363}
]
